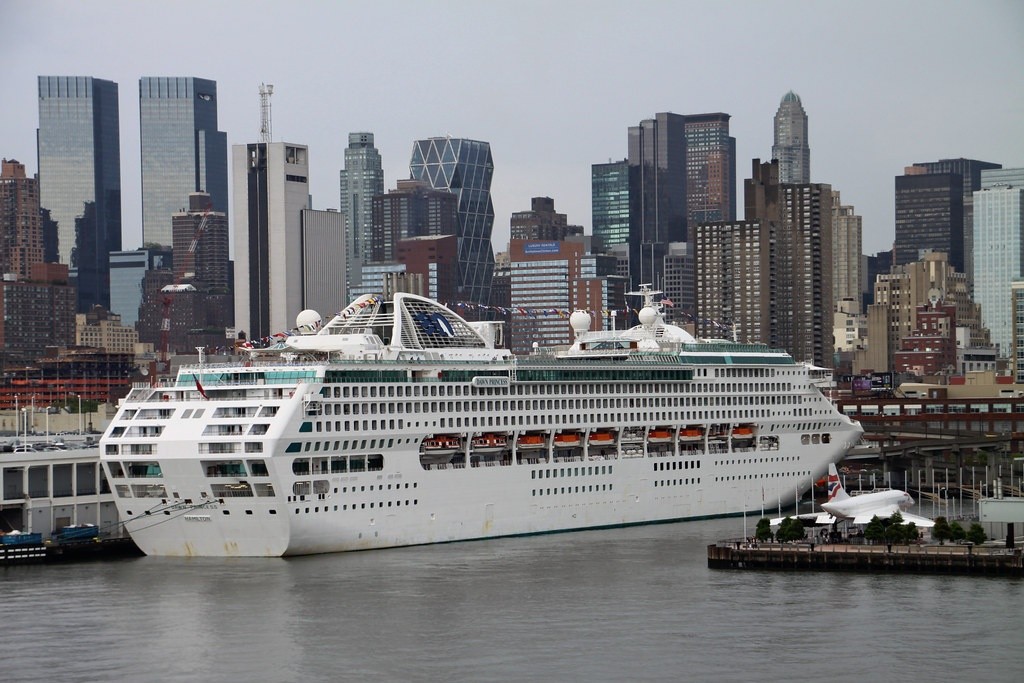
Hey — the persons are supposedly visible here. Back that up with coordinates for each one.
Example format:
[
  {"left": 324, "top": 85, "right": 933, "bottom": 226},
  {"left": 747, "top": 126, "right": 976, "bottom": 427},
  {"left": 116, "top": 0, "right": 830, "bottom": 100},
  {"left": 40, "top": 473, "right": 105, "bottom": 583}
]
[
  {"left": 747, "top": 537, "right": 756, "bottom": 547},
  {"left": 86, "top": 422, "right": 94, "bottom": 434},
  {"left": 810, "top": 535, "right": 815, "bottom": 551},
  {"left": 920, "top": 532, "right": 923, "bottom": 538}
]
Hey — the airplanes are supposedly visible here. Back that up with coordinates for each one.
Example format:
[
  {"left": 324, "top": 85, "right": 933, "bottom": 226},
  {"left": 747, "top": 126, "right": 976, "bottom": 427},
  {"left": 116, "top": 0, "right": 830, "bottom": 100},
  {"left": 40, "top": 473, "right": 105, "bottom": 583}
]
[{"left": 760, "top": 462, "right": 938, "bottom": 527}]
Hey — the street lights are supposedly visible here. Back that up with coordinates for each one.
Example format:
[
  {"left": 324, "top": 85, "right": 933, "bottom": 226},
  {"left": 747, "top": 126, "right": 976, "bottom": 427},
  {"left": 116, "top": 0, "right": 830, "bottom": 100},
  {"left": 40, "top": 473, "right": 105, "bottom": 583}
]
[
  {"left": 25, "top": 367, "right": 33, "bottom": 407},
  {"left": 45, "top": 405, "right": 52, "bottom": 444},
  {"left": 76, "top": 395, "right": 82, "bottom": 435},
  {"left": 31, "top": 395, "right": 35, "bottom": 435},
  {"left": 21, "top": 407, "right": 27, "bottom": 454},
  {"left": 13, "top": 395, "right": 19, "bottom": 437}
]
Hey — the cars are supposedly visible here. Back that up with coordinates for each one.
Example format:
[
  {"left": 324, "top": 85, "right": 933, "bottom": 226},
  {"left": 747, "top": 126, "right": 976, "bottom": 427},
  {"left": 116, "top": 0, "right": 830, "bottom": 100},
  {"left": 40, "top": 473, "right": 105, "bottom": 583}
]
[{"left": 14, "top": 441, "right": 67, "bottom": 454}]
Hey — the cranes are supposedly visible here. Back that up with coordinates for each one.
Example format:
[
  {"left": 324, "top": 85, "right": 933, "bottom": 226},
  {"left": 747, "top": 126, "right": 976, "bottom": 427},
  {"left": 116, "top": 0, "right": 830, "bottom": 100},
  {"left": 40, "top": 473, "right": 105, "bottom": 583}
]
[{"left": 158, "top": 201, "right": 216, "bottom": 364}]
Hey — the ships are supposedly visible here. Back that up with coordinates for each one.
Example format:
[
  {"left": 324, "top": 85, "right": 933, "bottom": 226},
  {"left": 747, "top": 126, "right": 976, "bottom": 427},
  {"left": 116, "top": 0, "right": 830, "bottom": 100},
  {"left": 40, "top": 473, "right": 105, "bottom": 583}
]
[{"left": 97, "top": 281, "right": 865, "bottom": 558}]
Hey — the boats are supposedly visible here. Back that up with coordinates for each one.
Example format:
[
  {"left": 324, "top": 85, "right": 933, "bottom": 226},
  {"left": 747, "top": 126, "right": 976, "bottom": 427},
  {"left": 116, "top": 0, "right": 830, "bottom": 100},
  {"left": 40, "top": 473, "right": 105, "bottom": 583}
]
[
  {"left": 588, "top": 433, "right": 616, "bottom": 445},
  {"left": 553, "top": 435, "right": 581, "bottom": 448},
  {"left": 678, "top": 429, "right": 702, "bottom": 442},
  {"left": 515, "top": 435, "right": 545, "bottom": 449},
  {"left": 647, "top": 431, "right": 673, "bottom": 444},
  {"left": 0, "top": 528, "right": 45, "bottom": 546},
  {"left": 470, "top": 434, "right": 507, "bottom": 453},
  {"left": 732, "top": 427, "right": 753, "bottom": 439},
  {"left": 423, "top": 434, "right": 460, "bottom": 455},
  {"left": 51, "top": 522, "right": 100, "bottom": 542}
]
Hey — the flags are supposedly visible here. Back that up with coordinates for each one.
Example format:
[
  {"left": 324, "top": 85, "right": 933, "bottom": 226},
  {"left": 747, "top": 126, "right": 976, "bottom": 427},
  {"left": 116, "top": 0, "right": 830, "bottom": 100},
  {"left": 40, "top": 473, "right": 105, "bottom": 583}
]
[
  {"left": 193, "top": 373, "right": 208, "bottom": 398},
  {"left": 242, "top": 295, "right": 383, "bottom": 347},
  {"left": 661, "top": 295, "right": 674, "bottom": 307},
  {"left": 457, "top": 301, "right": 634, "bottom": 319}
]
